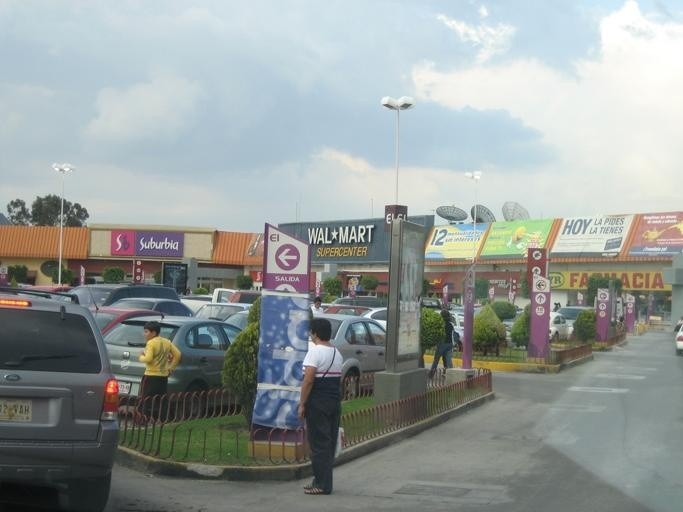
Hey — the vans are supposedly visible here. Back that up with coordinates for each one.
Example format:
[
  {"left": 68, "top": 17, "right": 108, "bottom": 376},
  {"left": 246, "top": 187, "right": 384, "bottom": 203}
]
[{"left": 0, "top": 286, "right": 119, "bottom": 511}]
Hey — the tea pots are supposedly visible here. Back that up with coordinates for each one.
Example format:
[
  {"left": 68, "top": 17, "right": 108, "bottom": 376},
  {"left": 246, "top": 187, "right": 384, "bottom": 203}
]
[{"left": 642, "top": 226, "right": 666, "bottom": 242}]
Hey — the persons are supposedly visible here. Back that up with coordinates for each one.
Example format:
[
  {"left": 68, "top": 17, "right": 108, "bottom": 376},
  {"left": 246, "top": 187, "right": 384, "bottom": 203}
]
[
  {"left": 138, "top": 321, "right": 182, "bottom": 425},
  {"left": 310, "top": 297, "right": 323, "bottom": 316},
  {"left": 553, "top": 300, "right": 571, "bottom": 312},
  {"left": 428, "top": 310, "right": 454, "bottom": 379},
  {"left": 298, "top": 318, "right": 343, "bottom": 495},
  {"left": 442, "top": 321, "right": 454, "bottom": 378}
]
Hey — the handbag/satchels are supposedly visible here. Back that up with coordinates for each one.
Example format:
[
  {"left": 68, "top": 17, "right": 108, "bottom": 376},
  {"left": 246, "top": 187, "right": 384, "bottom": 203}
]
[{"left": 296, "top": 403, "right": 307, "bottom": 417}]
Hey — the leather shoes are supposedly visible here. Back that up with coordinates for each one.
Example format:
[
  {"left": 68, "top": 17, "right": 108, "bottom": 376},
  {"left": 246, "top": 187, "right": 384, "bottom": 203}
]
[{"left": 303, "top": 481, "right": 330, "bottom": 495}]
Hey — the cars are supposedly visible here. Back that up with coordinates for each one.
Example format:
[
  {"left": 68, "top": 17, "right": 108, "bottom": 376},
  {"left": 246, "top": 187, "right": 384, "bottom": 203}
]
[
  {"left": 672, "top": 318, "right": 683, "bottom": 347},
  {"left": 311, "top": 313, "right": 386, "bottom": 400},
  {"left": 102, "top": 313, "right": 243, "bottom": 422},
  {"left": 675, "top": 322, "right": 683, "bottom": 355}
]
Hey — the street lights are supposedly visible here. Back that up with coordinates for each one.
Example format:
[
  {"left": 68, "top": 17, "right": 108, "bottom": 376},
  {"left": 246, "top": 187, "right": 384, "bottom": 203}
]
[
  {"left": 463, "top": 170, "right": 482, "bottom": 264},
  {"left": 50, "top": 163, "right": 73, "bottom": 286},
  {"left": 369, "top": 197, "right": 374, "bottom": 218},
  {"left": 380, "top": 95, "right": 416, "bottom": 220}
]
